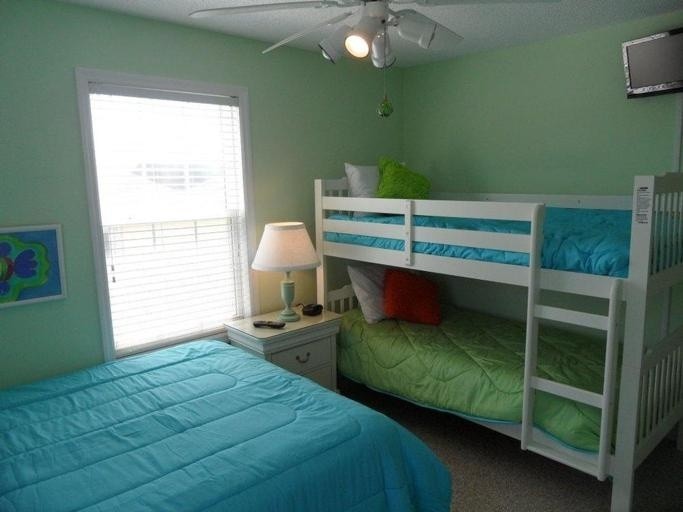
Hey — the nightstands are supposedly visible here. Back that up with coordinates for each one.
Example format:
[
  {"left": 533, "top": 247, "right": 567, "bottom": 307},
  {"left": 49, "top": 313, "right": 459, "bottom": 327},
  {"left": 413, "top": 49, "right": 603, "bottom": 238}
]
[{"left": 224, "top": 305, "right": 344, "bottom": 396}]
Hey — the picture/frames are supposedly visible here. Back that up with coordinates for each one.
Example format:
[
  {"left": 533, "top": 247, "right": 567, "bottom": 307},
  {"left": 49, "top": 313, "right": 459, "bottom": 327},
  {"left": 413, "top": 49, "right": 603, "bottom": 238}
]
[{"left": 0, "top": 223, "right": 67, "bottom": 310}]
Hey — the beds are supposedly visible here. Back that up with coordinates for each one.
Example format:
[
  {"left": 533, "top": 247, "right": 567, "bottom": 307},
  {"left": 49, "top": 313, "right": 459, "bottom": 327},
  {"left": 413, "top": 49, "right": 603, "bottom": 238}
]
[
  {"left": 311, "top": 176, "right": 683, "bottom": 511},
  {"left": 2, "top": 336, "right": 397, "bottom": 512}
]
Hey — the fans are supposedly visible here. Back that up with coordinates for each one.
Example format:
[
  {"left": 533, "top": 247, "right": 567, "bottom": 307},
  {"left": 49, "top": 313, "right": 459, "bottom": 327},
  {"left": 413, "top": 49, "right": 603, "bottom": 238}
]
[{"left": 185, "top": 0, "right": 481, "bottom": 56}]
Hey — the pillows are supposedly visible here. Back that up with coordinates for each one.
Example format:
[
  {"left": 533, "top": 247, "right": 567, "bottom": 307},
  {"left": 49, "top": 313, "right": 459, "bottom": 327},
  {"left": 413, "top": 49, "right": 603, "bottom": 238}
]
[
  {"left": 343, "top": 160, "right": 431, "bottom": 218},
  {"left": 346, "top": 259, "right": 441, "bottom": 327}
]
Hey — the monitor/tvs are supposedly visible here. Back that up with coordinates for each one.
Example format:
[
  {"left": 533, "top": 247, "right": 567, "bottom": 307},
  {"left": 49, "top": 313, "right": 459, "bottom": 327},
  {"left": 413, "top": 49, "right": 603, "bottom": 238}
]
[{"left": 619, "top": 26, "right": 683, "bottom": 100}]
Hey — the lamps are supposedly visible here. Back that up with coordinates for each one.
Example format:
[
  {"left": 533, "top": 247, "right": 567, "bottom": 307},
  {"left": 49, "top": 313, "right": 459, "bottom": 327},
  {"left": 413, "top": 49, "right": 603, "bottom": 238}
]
[
  {"left": 251, "top": 221, "right": 323, "bottom": 323},
  {"left": 315, "top": 1, "right": 443, "bottom": 71}
]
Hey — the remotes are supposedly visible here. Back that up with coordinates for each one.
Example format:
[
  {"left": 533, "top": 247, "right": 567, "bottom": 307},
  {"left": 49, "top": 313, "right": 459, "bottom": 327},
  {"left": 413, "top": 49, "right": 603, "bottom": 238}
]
[{"left": 253, "top": 319, "right": 286, "bottom": 330}]
